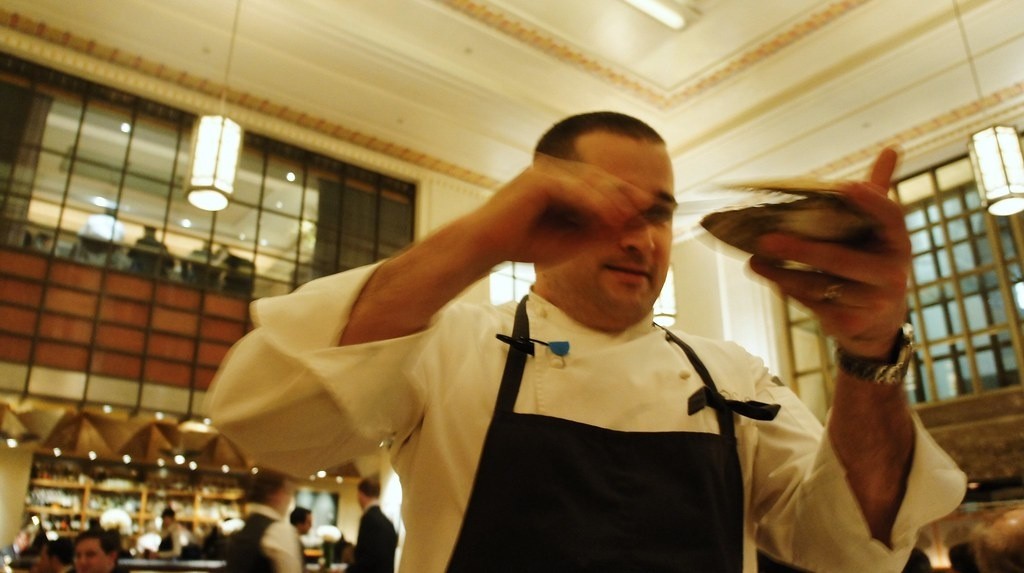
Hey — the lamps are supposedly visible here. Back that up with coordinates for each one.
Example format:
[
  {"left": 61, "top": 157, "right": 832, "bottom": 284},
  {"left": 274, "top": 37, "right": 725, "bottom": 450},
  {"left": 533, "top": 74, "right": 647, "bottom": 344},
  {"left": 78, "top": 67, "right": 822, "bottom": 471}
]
[
  {"left": 953, "top": 0, "right": 1024, "bottom": 216},
  {"left": 182, "top": 0, "right": 247, "bottom": 212}
]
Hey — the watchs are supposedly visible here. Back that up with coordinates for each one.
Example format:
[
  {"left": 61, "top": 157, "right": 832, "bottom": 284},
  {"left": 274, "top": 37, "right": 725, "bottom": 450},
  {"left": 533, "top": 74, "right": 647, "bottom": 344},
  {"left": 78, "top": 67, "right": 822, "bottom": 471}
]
[{"left": 833, "top": 322, "right": 918, "bottom": 385}]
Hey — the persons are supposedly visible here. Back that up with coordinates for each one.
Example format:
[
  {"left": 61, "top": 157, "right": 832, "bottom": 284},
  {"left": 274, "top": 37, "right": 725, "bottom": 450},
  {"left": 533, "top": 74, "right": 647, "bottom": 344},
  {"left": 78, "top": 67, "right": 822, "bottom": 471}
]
[
  {"left": 128, "top": 225, "right": 176, "bottom": 282},
  {"left": 201, "top": 111, "right": 969, "bottom": 573},
  {"left": 22, "top": 225, "right": 58, "bottom": 256},
  {"left": 76, "top": 204, "right": 127, "bottom": 269},
  {"left": 144, "top": 509, "right": 184, "bottom": 560},
  {"left": 902, "top": 548, "right": 933, "bottom": 573},
  {"left": 226, "top": 466, "right": 305, "bottom": 573},
  {"left": 290, "top": 506, "right": 315, "bottom": 573},
  {"left": 181, "top": 239, "right": 225, "bottom": 286},
  {"left": 0, "top": 513, "right": 133, "bottom": 568},
  {"left": 73, "top": 529, "right": 129, "bottom": 573},
  {"left": 39, "top": 539, "right": 76, "bottom": 573},
  {"left": 948, "top": 541, "right": 982, "bottom": 573},
  {"left": 342, "top": 476, "right": 398, "bottom": 573}
]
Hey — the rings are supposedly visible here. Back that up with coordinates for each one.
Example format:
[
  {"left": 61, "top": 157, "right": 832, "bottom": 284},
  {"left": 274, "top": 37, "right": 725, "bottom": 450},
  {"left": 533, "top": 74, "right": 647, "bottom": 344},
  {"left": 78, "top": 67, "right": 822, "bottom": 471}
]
[{"left": 823, "top": 276, "right": 844, "bottom": 304}]
